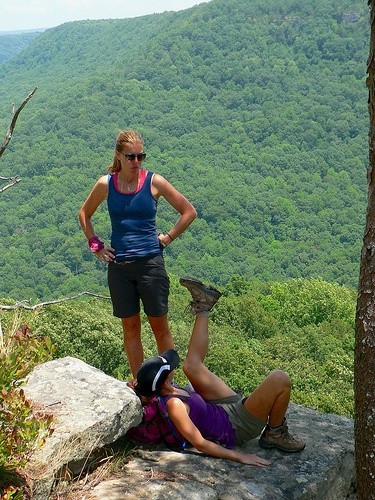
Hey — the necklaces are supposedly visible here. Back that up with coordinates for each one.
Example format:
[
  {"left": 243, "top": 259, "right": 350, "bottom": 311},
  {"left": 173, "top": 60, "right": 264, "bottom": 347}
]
[{"left": 120, "top": 170, "right": 140, "bottom": 192}]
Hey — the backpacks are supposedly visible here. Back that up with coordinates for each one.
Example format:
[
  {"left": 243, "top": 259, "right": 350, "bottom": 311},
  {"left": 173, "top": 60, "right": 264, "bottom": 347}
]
[{"left": 126, "top": 395, "right": 185, "bottom": 452}]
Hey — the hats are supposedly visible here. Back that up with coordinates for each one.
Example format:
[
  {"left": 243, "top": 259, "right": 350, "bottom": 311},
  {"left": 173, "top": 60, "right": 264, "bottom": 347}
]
[{"left": 136, "top": 349, "right": 179, "bottom": 397}]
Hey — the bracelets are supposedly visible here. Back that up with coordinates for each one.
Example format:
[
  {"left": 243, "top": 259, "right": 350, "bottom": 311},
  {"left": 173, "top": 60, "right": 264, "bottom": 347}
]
[
  {"left": 167, "top": 233, "right": 174, "bottom": 242},
  {"left": 88, "top": 236, "right": 105, "bottom": 253}
]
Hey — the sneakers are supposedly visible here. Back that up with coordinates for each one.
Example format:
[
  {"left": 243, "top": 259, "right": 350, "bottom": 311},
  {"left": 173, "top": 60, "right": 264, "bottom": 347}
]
[
  {"left": 179, "top": 277, "right": 221, "bottom": 315},
  {"left": 259, "top": 417, "right": 305, "bottom": 453}
]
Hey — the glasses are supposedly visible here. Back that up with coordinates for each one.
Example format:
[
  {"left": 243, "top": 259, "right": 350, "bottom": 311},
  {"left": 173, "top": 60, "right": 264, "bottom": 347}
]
[{"left": 117, "top": 149, "right": 146, "bottom": 161}]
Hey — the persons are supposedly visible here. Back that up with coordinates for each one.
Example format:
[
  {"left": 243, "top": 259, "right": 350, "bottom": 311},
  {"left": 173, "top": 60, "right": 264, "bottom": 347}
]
[
  {"left": 78, "top": 129, "right": 203, "bottom": 387},
  {"left": 135, "top": 273, "right": 307, "bottom": 468}
]
